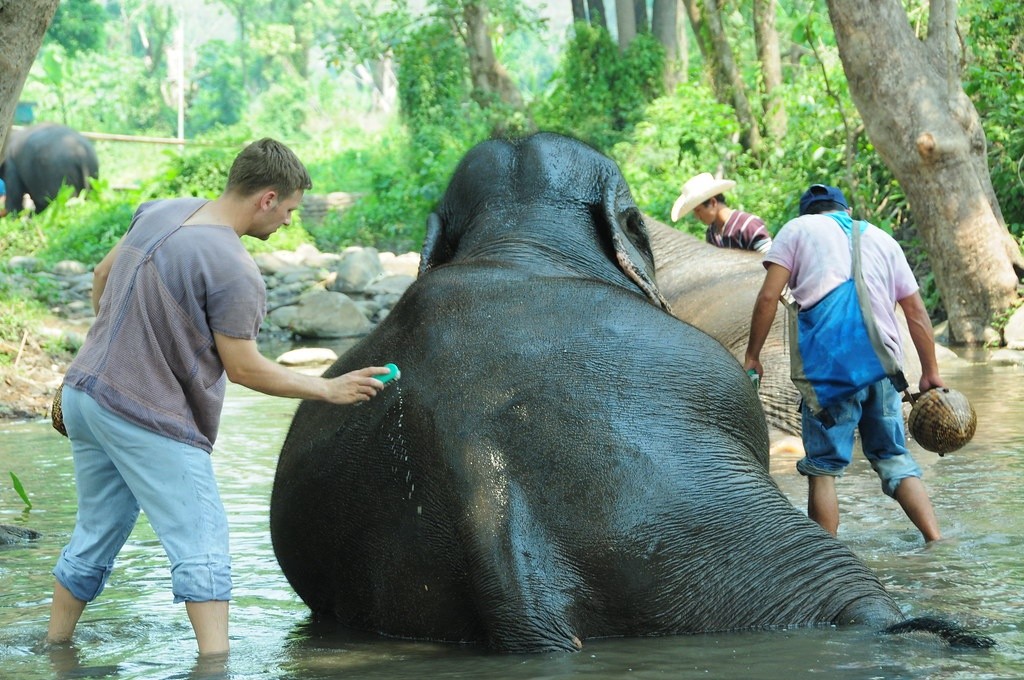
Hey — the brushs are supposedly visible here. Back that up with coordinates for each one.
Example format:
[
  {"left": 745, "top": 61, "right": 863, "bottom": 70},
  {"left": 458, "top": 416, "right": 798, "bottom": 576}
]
[
  {"left": 747, "top": 370, "right": 760, "bottom": 393},
  {"left": 352, "top": 364, "right": 402, "bottom": 407}
]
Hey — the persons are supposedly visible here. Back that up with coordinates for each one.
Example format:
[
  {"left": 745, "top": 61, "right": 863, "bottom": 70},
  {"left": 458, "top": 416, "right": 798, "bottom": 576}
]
[
  {"left": 48, "top": 137, "right": 390, "bottom": 654},
  {"left": 671, "top": 173, "right": 772, "bottom": 254},
  {"left": 743, "top": 185, "right": 943, "bottom": 541}
]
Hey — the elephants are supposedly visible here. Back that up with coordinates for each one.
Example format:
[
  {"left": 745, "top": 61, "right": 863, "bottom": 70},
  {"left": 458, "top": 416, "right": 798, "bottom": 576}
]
[
  {"left": 269, "top": 130, "right": 1000, "bottom": 657},
  {"left": 0, "top": 120, "right": 100, "bottom": 220}
]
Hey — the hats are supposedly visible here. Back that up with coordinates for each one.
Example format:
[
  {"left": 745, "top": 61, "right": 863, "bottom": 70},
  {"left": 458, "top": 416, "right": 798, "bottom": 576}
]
[
  {"left": 799, "top": 184, "right": 848, "bottom": 216},
  {"left": 670, "top": 173, "right": 736, "bottom": 221}
]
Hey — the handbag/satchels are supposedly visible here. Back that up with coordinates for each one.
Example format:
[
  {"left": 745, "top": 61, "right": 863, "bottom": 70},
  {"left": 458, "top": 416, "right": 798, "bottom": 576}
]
[{"left": 786, "top": 278, "right": 902, "bottom": 416}]
